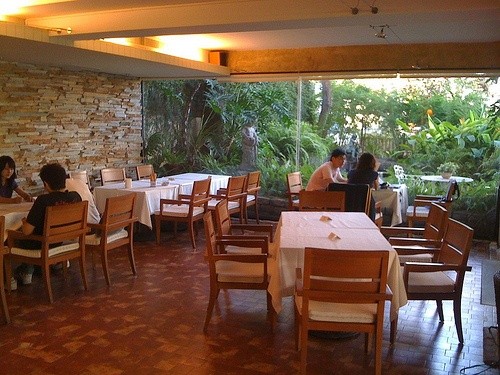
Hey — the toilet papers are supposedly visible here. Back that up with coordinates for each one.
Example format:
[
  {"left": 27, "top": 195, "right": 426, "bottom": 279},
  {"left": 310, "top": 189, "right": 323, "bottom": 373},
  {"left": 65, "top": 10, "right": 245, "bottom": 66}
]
[{"left": 125, "top": 178, "right": 132, "bottom": 189}]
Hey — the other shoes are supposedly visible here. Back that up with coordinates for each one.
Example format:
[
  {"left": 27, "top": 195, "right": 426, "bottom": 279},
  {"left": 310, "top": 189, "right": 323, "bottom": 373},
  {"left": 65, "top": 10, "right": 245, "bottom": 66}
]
[
  {"left": 20, "top": 272, "right": 32, "bottom": 283},
  {"left": 4, "top": 280, "right": 17, "bottom": 290}
]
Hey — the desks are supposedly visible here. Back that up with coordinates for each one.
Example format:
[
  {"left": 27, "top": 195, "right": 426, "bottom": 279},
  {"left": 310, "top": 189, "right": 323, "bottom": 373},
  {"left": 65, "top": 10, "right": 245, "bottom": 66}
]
[
  {"left": 420, "top": 175, "right": 474, "bottom": 195},
  {"left": 141, "top": 172, "right": 232, "bottom": 201},
  {"left": 273, "top": 211, "right": 380, "bottom": 241},
  {"left": 0, "top": 196, "right": 38, "bottom": 244},
  {"left": 267, "top": 226, "right": 408, "bottom": 340},
  {"left": 93, "top": 181, "right": 179, "bottom": 242},
  {"left": 369, "top": 183, "right": 408, "bottom": 227}
]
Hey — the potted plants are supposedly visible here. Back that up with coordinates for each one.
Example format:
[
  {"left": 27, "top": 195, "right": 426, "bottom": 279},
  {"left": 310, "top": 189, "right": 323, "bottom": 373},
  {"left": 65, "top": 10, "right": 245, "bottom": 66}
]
[{"left": 437, "top": 161, "right": 460, "bottom": 179}]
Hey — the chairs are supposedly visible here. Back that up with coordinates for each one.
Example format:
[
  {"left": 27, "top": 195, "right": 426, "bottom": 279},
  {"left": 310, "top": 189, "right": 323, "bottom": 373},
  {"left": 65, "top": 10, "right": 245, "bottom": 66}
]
[{"left": 0, "top": 164, "right": 474, "bottom": 375}]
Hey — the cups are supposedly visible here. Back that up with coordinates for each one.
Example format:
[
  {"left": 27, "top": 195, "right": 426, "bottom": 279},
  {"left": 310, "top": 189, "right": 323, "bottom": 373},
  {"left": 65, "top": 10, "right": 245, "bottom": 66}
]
[{"left": 124, "top": 178, "right": 132, "bottom": 189}]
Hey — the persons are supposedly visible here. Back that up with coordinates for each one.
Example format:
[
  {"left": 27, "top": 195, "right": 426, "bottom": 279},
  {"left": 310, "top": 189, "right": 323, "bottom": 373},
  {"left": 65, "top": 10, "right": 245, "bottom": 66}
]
[
  {"left": 344, "top": 153, "right": 380, "bottom": 224},
  {"left": 307, "top": 149, "right": 349, "bottom": 210},
  {"left": 0, "top": 155, "right": 100, "bottom": 293}
]
